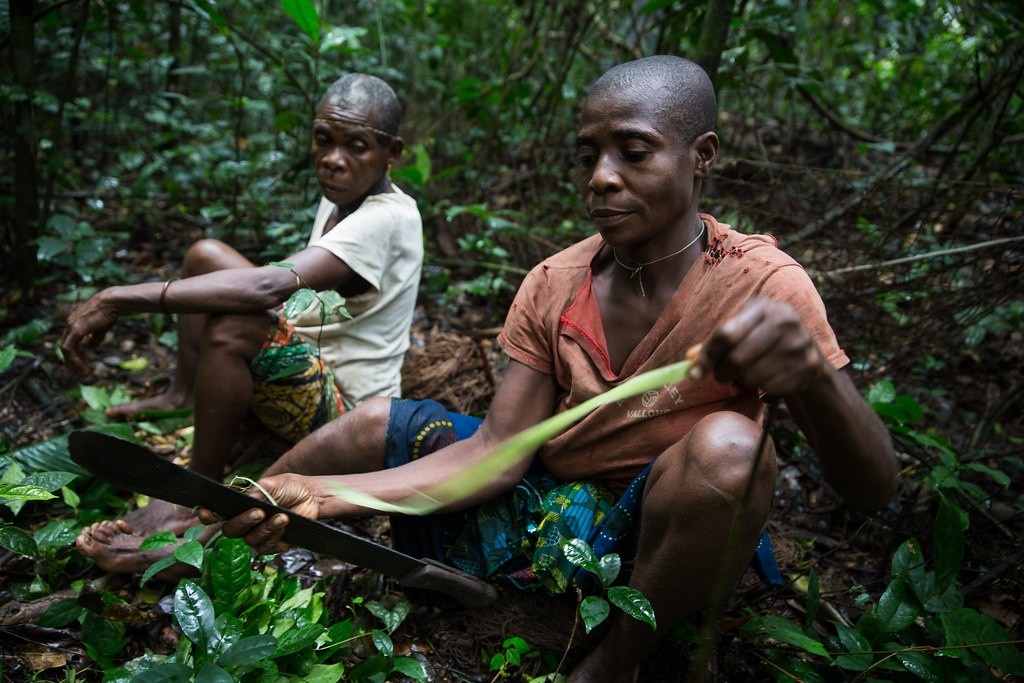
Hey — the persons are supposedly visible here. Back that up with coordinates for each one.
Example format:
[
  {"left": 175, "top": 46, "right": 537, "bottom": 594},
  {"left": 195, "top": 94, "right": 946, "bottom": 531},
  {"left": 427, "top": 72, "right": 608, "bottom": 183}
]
[
  {"left": 61, "top": 73, "right": 426, "bottom": 539},
  {"left": 76, "top": 56, "right": 897, "bottom": 683}
]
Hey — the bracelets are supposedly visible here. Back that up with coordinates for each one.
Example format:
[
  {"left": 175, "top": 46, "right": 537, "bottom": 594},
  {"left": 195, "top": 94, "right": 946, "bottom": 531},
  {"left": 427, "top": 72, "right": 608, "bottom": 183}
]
[{"left": 159, "top": 279, "right": 177, "bottom": 314}]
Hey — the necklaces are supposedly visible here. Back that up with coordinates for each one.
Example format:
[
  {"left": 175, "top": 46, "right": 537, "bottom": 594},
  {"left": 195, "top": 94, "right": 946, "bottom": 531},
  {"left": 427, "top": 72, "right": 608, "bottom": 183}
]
[{"left": 613, "top": 219, "right": 707, "bottom": 297}]
